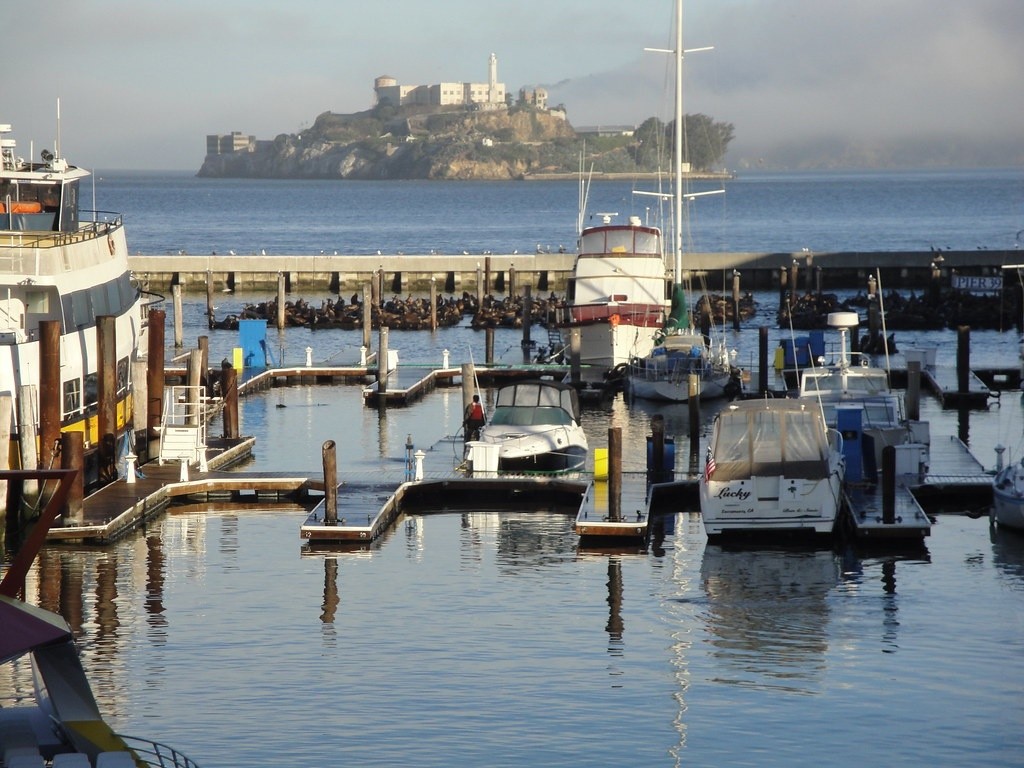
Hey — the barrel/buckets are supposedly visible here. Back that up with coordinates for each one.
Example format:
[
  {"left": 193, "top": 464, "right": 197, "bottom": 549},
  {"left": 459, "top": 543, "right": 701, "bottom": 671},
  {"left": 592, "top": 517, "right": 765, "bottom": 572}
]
[{"left": 645, "top": 435, "right": 675, "bottom": 472}]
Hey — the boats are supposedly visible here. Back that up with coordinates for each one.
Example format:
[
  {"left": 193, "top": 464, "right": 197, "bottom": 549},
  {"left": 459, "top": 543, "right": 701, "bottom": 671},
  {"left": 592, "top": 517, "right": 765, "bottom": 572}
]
[
  {"left": 691, "top": 342, "right": 849, "bottom": 543},
  {"left": 991, "top": 457, "right": 1024, "bottom": 534},
  {"left": 0, "top": 95, "right": 166, "bottom": 536},
  {"left": 461, "top": 347, "right": 588, "bottom": 476},
  {"left": 548, "top": 137, "right": 672, "bottom": 374},
  {"left": 774, "top": 267, "right": 933, "bottom": 480}
]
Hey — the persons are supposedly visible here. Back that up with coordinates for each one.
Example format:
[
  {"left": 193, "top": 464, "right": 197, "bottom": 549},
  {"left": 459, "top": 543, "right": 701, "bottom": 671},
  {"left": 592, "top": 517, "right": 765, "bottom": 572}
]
[{"left": 464, "top": 395, "right": 486, "bottom": 443}]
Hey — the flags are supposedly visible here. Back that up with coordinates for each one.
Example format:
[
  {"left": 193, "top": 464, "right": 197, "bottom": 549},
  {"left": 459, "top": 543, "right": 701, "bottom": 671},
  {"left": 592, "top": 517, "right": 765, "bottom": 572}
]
[{"left": 704, "top": 446, "right": 714, "bottom": 483}]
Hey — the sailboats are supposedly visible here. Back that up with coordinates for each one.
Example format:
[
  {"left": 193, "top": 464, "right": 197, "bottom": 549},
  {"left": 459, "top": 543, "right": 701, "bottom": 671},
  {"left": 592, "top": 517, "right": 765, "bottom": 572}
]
[{"left": 613, "top": 0, "right": 734, "bottom": 405}]
